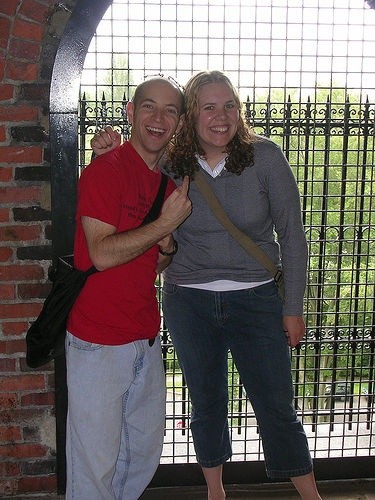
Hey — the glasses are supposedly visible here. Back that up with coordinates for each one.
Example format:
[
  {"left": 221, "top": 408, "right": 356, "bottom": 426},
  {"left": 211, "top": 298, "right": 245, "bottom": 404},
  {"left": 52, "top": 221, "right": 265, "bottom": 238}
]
[{"left": 143, "top": 72, "right": 181, "bottom": 88}]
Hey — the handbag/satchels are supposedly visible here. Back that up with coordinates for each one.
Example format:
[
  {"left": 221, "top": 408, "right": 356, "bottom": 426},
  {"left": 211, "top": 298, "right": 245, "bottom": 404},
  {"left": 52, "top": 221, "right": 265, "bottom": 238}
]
[{"left": 26, "top": 261, "right": 97, "bottom": 369}]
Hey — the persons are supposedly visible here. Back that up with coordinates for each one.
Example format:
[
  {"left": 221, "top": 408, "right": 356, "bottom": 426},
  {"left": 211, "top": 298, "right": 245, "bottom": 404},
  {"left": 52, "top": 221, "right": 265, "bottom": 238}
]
[
  {"left": 65, "top": 77, "right": 192, "bottom": 500},
  {"left": 90, "top": 71, "right": 323, "bottom": 500}
]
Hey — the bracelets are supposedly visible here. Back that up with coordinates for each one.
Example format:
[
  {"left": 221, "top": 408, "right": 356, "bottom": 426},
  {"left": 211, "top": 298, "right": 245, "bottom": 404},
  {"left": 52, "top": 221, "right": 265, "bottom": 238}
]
[{"left": 158, "top": 241, "right": 179, "bottom": 256}]
